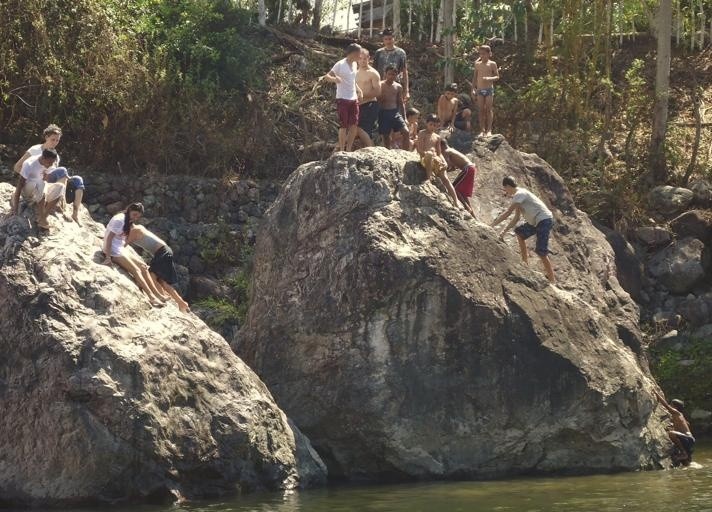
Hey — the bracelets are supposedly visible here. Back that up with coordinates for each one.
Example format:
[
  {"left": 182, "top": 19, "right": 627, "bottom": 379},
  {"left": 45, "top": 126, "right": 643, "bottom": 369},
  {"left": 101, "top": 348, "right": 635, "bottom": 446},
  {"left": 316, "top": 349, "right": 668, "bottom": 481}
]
[{"left": 105, "top": 256, "right": 111, "bottom": 259}]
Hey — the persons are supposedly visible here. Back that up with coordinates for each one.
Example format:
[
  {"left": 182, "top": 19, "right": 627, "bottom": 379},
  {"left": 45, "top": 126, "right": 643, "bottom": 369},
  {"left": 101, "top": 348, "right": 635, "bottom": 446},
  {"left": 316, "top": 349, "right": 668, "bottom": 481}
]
[
  {"left": 470, "top": 44, "right": 500, "bottom": 139},
  {"left": 490, "top": 175, "right": 556, "bottom": 284},
  {"left": 100, "top": 203, "right": 171, "bottom": 306},
  {"left": 323, "top": 29, "right": 421, "bottom": 155},
  {"left": 15, "top": 125, "right": 84, "bottom": 228},
  {"left": 416, "top": 113, "right": 465, "bottom": 211},
  {"left": 123, "top": 224, "right": 188, "bottom": 312},
  {"left": 437, "top": 82, "right": 472, "bottom": 134},
  {"left": 440, "top": 138, "right": 477, "bottom": 220},
  {"left": 651, "top": 386, "right": 696, "bottom": 467},
  {"left": 10, "top": 148, "right": 62, "bottom": 229}
]
